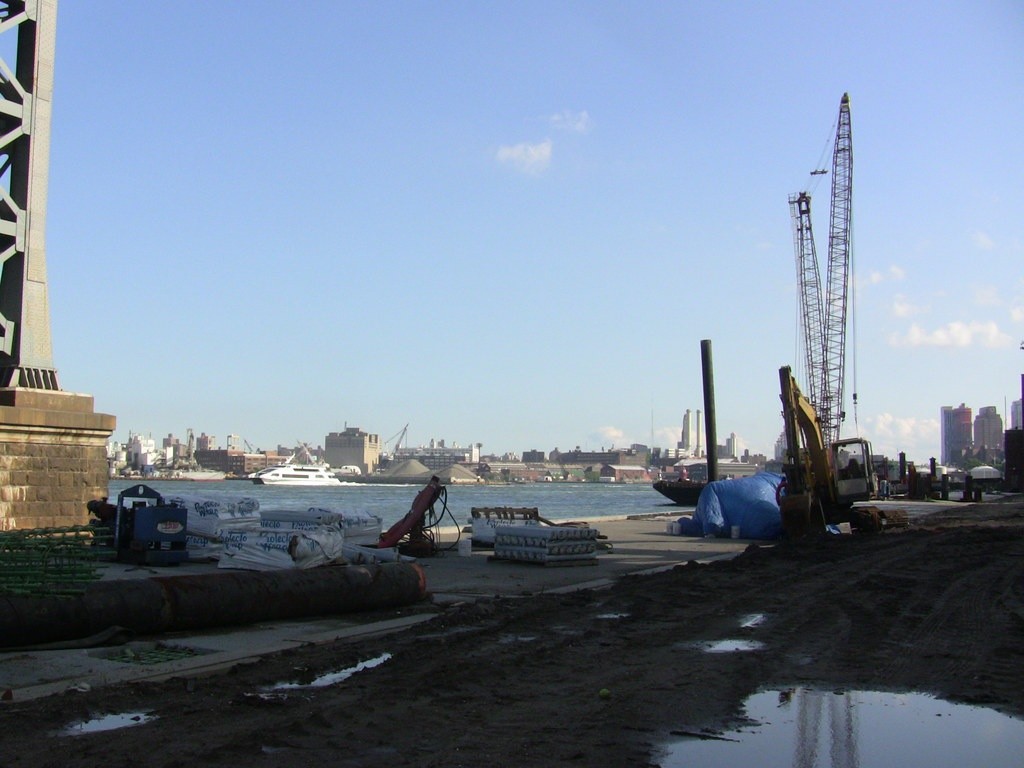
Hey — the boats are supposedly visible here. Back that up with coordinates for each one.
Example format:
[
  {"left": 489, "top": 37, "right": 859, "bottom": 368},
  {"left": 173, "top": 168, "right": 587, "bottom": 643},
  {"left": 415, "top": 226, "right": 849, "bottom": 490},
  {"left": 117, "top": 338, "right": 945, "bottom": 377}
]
[
  {"left": 652, "top": 454, "right": 764, "bottom": 507},
  {"left": 246, "top": 440, "right": 343, "bottom": 486}
]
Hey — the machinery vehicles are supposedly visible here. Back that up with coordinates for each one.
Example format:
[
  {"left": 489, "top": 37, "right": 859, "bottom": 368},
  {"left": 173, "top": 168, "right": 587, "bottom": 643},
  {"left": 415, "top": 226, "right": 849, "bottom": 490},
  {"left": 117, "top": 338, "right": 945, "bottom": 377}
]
[{"left": 777, "top": 365, "right": 910, "bottom": 556}]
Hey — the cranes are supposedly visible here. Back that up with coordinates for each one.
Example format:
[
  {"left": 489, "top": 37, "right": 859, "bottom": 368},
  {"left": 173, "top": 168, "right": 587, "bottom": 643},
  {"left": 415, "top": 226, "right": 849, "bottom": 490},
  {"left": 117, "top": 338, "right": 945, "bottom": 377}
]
[
  {"left": 384, "top": 421, "right": 410, "bottom": 459},
  {"left": 786, "top": 90, "right": 858, "bottom": 453}
]
